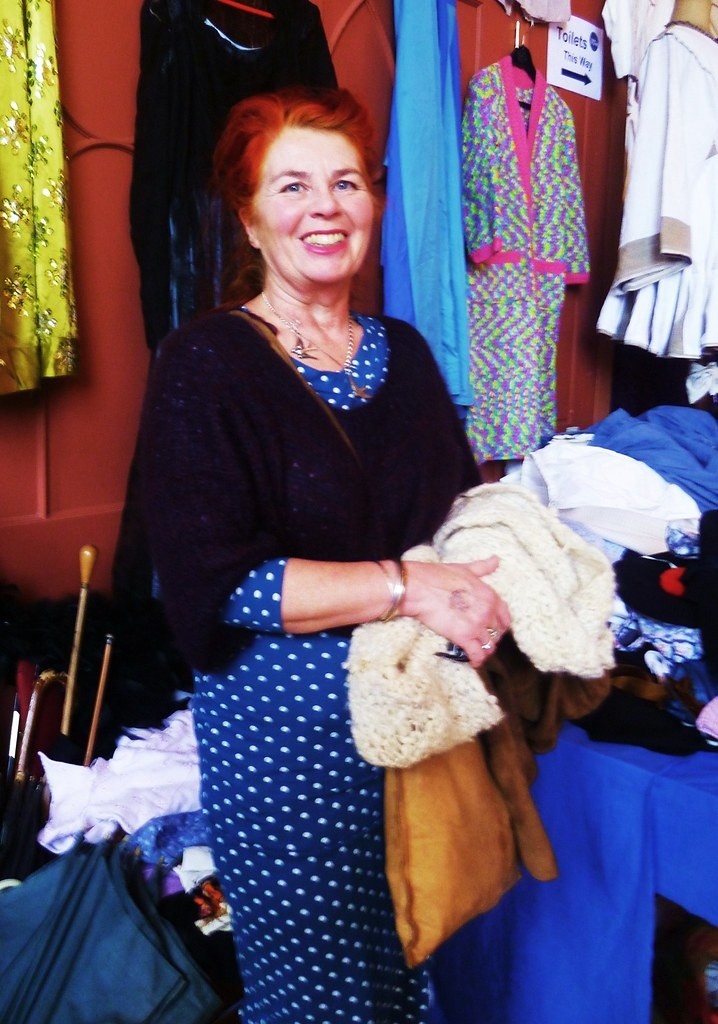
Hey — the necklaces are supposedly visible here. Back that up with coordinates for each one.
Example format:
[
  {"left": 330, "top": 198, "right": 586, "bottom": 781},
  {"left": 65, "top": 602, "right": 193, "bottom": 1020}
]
[{"left": 262, "top": 293, "right": 371, "bottom": 398}]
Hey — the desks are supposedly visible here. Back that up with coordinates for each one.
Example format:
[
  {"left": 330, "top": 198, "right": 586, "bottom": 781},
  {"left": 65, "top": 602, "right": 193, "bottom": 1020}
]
[{"left": 491, "top": 725, "right": 718, "bottom": 1024}]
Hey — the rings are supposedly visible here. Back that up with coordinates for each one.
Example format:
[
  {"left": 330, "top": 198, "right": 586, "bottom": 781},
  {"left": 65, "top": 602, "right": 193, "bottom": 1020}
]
[
  {"left": 481, "top": 640, "right": 492, "bottom": 650},
  {"left": 488, "top": 628, "right": 499, "bottom": 639}
]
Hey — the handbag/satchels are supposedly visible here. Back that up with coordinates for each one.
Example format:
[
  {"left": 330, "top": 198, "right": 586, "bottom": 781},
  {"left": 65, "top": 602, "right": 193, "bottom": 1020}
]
[{"left": 386, "top": 718, "right": 552, "bottom": 968}]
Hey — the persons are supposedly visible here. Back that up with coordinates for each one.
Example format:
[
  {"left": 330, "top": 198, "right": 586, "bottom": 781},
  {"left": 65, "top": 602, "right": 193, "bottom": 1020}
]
[{"left": 106, "top": 85, "right": 507, "bottom": 1024}]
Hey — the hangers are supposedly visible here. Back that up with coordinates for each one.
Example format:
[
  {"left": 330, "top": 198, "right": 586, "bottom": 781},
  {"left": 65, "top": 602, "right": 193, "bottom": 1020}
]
[{"left": 512, "top": 14, "right": 538, "bottom": 80}]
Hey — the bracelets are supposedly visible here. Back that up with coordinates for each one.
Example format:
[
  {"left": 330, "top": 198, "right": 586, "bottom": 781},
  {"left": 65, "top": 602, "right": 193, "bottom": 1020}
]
[{"left": 375, "top": 557, "right": 405, "bottom": 623}]
